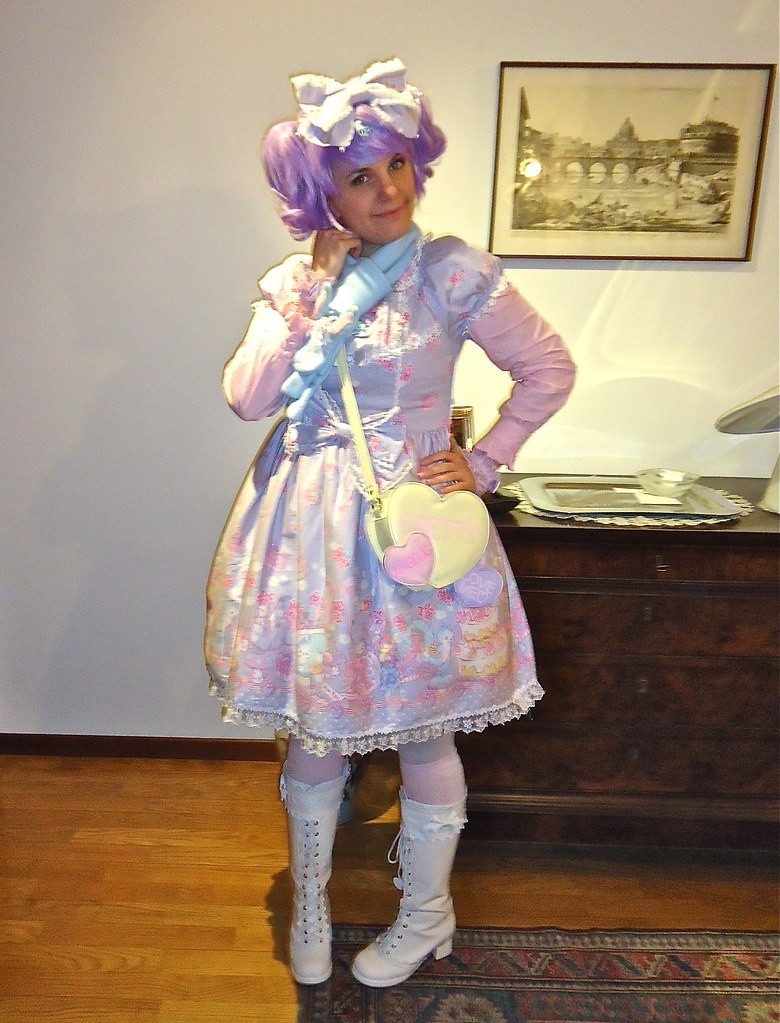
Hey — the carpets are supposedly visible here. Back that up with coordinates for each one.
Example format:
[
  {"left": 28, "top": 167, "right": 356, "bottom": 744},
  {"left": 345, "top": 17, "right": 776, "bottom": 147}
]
[{"left": 305, "top": 922, "right": 780, "bottom": 1023}]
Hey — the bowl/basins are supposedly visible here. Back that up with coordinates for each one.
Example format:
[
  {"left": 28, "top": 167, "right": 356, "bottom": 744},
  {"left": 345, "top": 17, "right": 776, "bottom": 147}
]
[{"left": 635, "top": 467, "right": 700, "bottom": 498}]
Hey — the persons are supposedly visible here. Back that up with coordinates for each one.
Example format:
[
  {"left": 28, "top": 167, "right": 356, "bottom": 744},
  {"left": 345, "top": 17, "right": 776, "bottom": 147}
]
[{"left": 204, "top": 58, "right": 578, "bottom": 987}]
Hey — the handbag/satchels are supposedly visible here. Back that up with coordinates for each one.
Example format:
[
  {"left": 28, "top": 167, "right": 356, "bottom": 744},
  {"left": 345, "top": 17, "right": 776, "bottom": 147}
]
[{"left": 362, "top": 480, "right": 490, "bottom": 591}]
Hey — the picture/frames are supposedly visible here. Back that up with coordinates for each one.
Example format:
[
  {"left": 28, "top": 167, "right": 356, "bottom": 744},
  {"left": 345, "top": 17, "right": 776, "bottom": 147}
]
[
  {"left": 488, "top": 61, "right": 777, "bottom": 262},
  {"left": 449, "top": 405, "right": 474, "bottom": 451}
]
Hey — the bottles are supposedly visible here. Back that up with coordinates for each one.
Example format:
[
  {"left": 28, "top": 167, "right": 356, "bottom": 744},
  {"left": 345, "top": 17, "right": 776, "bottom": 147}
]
[{"left": 451, "top": 406, "right": 475, "bottom": 450}]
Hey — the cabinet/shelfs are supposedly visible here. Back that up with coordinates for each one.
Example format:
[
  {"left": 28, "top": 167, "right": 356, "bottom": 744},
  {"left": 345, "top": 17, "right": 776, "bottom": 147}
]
[{"left": 454, "top": 474, "right": 779, "bottom": 853}]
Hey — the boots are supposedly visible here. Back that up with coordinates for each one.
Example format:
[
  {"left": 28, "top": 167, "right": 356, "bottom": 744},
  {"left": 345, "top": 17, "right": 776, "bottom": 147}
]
[
  {"left": 276, "top": 762, "right": 349, "bottom": 985},
  {"left": 347, "top": 789, "right": 467, "bottom": 989}
]
